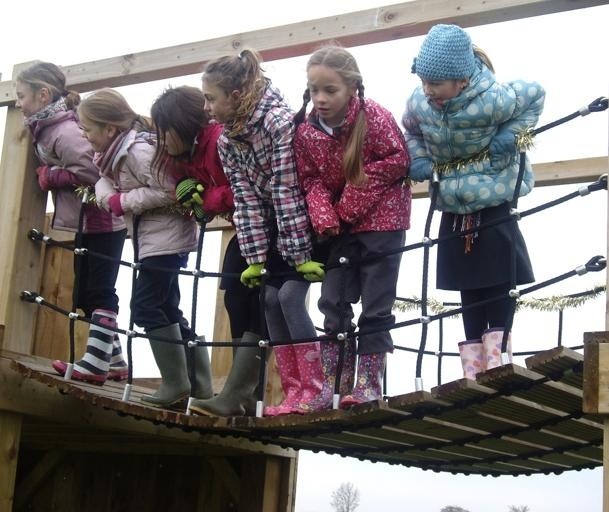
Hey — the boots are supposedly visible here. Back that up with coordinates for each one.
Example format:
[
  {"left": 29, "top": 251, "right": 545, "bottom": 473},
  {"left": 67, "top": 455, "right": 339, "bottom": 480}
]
[
  {"left": 52, "top": 308, "right": 117, "bottom": 386},
  {"left": 188, "top": 331, "right": 387, "bottom": 417},
  {"left": 107, "top": 321, "right": 128, "bottom": 381},
  {"left": 141, "top": 322, "right": 192, "bottom": 409},
  {"left": 170, "top": 335, "right": 214, "bottom": 408}
]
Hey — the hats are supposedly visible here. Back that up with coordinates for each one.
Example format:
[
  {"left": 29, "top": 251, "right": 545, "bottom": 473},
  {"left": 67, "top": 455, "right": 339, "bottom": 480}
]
[{"left": 411, "top": 23, "right": 475, "bottom": 80}]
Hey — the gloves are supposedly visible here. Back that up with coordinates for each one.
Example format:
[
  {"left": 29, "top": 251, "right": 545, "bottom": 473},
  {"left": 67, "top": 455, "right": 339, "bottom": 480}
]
[
  {"left": 108, "top": 192, "right": 125, "bottom": 217},
  {"left": 409, "top": 157, "right": 433, "bottom": 183},
  {"left": 240, "top": 261, "right": 266, "bottom": 289},
  {"left": 175, "top": 175, "right": 205, "bottom": 208},
  {"left": 488, "top": 132, "right": 515, "bottom": 157},
  {"left": 193, "top": 202, "right": 214, "bottom": 223},
  {"left": 295, "top": 259, "right": 326, "bottom": 282},
  {"left": 34, "top": 164, "right": 49, "bottom": 193}
]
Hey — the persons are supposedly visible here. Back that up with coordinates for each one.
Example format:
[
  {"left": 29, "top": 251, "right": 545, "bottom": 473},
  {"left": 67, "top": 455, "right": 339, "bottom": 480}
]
[
  {"left": 149, "top": 85, "right": 273, "bottom": 415},
  {"left": 291, "top": 46, "right": 413, "bottom": 409},
  {"left": 201, "top": 48, "right": 331, "bottom": 417},
  {"left": 15, "top": 61, "right": 129, "bottom": 386},
  {"left": 400, "top": 23, "right": 547, "bottom": 383},
  {"left": 76, "top": 86, "right": 215, "bottom": 409}
]
